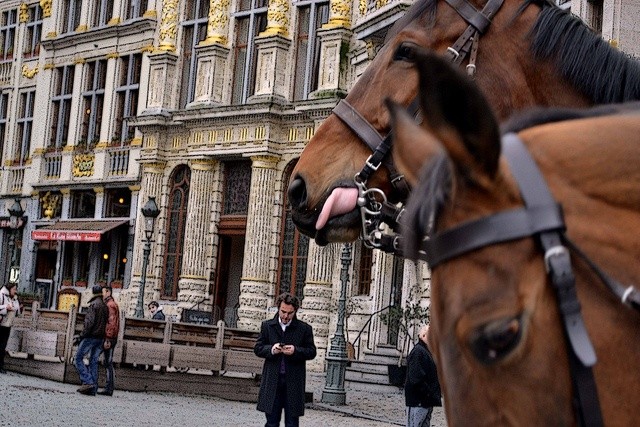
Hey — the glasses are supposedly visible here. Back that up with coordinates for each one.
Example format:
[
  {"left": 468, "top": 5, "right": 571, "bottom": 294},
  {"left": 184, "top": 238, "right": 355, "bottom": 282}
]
[{"left": 149, "top": 306, "right": 154, "bottom": 309}]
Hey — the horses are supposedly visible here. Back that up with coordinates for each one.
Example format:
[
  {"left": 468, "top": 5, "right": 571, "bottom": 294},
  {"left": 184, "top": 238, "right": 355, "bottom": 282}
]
[
  {"left": 389, "top": 44, "right": 639, "bottom": 427},
  {"left": 282, "top": 0, "right": 640, "bottom": 249}
]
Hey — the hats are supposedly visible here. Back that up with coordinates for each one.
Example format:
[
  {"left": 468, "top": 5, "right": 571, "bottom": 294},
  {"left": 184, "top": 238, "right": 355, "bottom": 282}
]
[{"left": 92, "top": 285, "right": 102, "bottom": 294}]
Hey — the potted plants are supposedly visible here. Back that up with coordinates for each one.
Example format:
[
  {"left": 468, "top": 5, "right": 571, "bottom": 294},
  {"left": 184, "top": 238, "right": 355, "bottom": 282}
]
[{"left": 377, "top": 283, "right": 430, "bottom": 387}]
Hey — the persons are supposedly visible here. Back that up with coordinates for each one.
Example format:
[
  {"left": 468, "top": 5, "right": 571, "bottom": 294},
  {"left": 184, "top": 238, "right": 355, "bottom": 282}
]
[
  {"left": 148, "top": 301, "right": 165, "bottom": 320},
  {"left": 404, "top": 326, "right": 442, "bottom": 427},
  {"left": 0, "top": 283, "right": 20, "bottom": 373},
  {"left": 97, "top": 286, "right": 120, "bottom": 396},
  {"left": 73, "top": 286, "right": 109, "bottom": 396},
  {"left": 254, "top": 292, "right": 316, "bottom": 427}
]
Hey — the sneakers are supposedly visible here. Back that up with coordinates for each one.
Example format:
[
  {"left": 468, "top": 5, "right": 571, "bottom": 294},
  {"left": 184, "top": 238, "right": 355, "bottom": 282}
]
[
  {"left": 98, "top": 390, "right": 113, "bottom": 396},
  {"left": 77, "top": 385, "right": 97, "bottom": 396}
]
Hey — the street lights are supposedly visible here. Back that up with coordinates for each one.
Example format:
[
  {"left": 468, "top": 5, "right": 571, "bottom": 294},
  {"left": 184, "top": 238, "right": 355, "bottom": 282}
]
[
  {"left": 130, "top": 195, "right": 161, "bottom": 318},
  {"left": 6, "top": 197, "right": 28, "bottom": 291}
]
